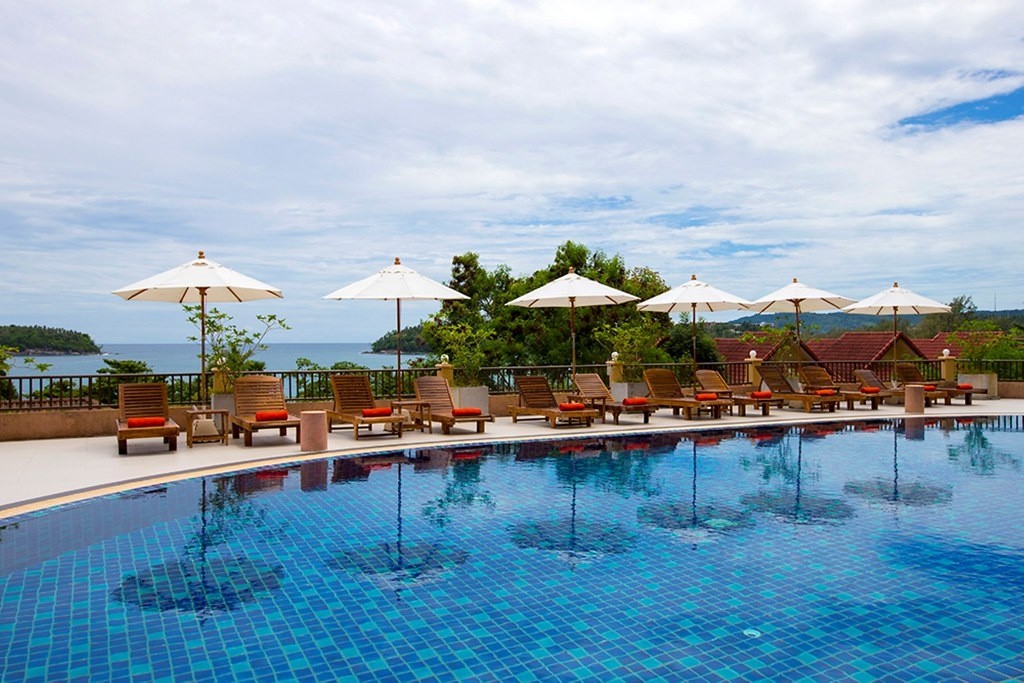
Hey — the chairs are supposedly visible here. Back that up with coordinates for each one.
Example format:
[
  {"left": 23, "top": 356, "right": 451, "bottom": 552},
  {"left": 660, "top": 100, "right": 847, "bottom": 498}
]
[
  {"left": 895, "top": 419, "right": 905, "bottom": 436},
  {"left": 506, "top": 376, "right": 600, "bottom": 429},
  {"left": 802, "top": 428, "right": 826, "bottom": 439},
  {"left": 752, "top": 365, "right": 846, "bottom": 412},
  {"left": 896, "top": 364, "right": 988, "bottom": 404},
  {"left": 651, "top": 433, "right": 681, "bottom": 454},
  {"left": 412, "top": 375, "right": 498, "bottom": 435},
  {"left": 513, "top": 441, "right": 553, "bottom": 462},
  {"left": 694, "top": 370, "right": 784, "bottom": 416},
  {"left": 642, "top": 368, "right": 735, "bottom": 422},
  {"left": 235, "top": 472, "right": 284, "bottom": 493},
  {"left": 799, "top": 365, "right": 893, "bottom": 410},
  {"left": 114, "top": 383, "right": 180, "bottom": 455},
  {"left": 413, "top": 449, "right": 452, "bottom": 474},
  {"left": 324, "top": 375, "right": 408, "bottom": 441},
  {"left": 568, "top": 373, "right": 658, "bottom": 426},
  {"left": 228, "top": 374, "right": 302, "bottom": 447},
  {"left": 331, "top": 458, "right": 371, "bottom": 485},
  {"left": 757, "top": 426, "right": 792, "bottom": 448},
  {"left": 852, "top": 369, "right": 948, "bottom": 407}
]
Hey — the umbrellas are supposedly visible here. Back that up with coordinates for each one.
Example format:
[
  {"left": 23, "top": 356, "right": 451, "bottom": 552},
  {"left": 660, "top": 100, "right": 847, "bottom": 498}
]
[
  {"left": 840, "top": 281, "right": 952, "bottom": 382},
  {"left": 110, "top": 477, "right": 286, "bottom": 628},
  {"left": 109, "top": 249, "right": 285, "bottom": 420},
  {"left": 750, "top": 277, "right": 859, "bottom": 384},
  {"left": 635, "top": 442, "right": 757, "bottom": 551},
  {"left": 636, "top": 275, "right": 756, "bottom": 399},
  {"left": 323, "top": 464, "right": 469, "bottom": 601},
  {"left": 505, "top": 265, "right": 641, "bottom": 395},
  {"left": 320, "top": 256, "right": 471, "bottom": 402},
  {"left": 843, "top": 430, "right": 955, "bottom": 520},
  {"left": 507, "top": 481, "right": 638, "bottom": 573},
  {"left": 740, "top": 433, "right": 859, "bottom": 530}
]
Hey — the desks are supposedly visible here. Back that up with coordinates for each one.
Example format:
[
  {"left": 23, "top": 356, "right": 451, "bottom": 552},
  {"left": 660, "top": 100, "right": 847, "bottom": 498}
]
[
  {"left": 697, "top": 390, "right": 733, "bottom": 418},
  {"left": 802, "top": 384, "right": 841, "bottom": 409},
  {"left": 568, "top": 395, "right": 608, "bottom": 424},
  {"left": 390, "top": 401, "right": 433, "bottom": 437},
  {"left": 185, "top": 409, "right": 229, "bottom": 448}
]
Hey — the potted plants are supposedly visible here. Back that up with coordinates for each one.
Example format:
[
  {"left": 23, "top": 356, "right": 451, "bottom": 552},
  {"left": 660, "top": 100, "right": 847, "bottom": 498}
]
[
  {"left": 182, "top": 477, "right": 290, "bottom": 572},
  {"left": 948, "top": 416, "right": 1023, "bottom": 477},
  {"left": 425, "top": 312, "right": 493, "bottom": 419},
  {"left": 946, "top": 318, "right": 999, "bottom": 399},
  {"left": 421, "top": 458, "right": 496, "bottom": 526},
  {"left": 181, "top": 304, "right": 292, "bottom": 434},
  {"left": 760, "top": 434, "right": 798, "bottom": 486},
  {"left": 596, "top": 451, "right": 665, "bottom": 500},
  {"left": 591, "top": 316, "right": 663, "bottom": 416},
  {"left": 738, "top": 314, "right": 822, "bottom": 404}
]
